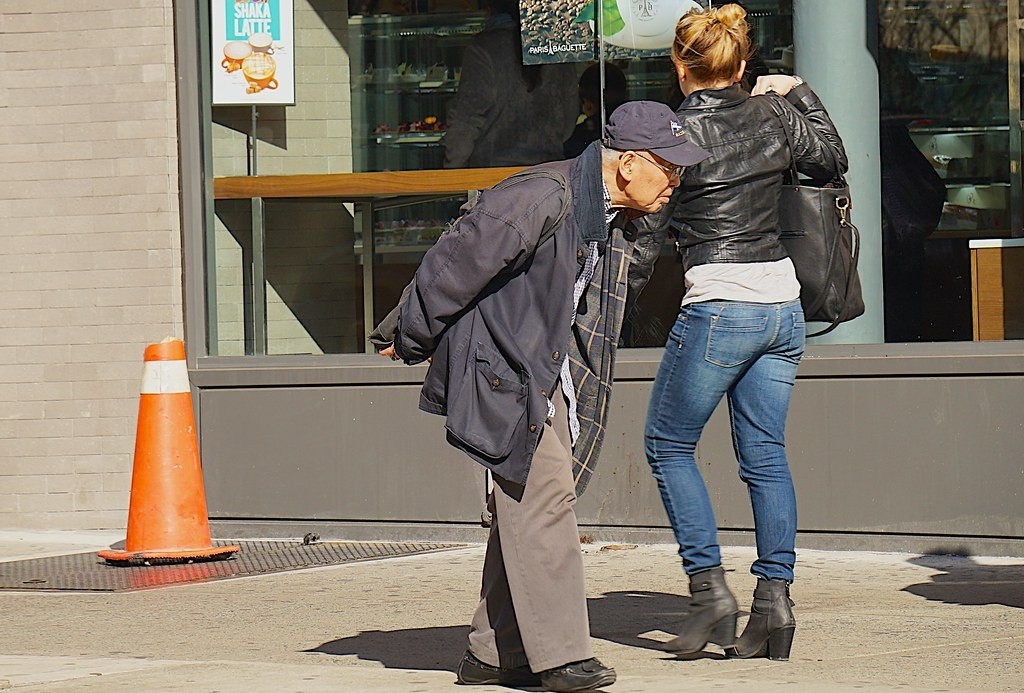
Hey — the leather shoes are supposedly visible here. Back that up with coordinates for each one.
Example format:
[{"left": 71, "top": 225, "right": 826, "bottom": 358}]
[
  {"left": 540, "top": 656, "right": 617, "bottom": 692},
  {"left": 456, "top": 650, "right": 542, "bottom": 688}
]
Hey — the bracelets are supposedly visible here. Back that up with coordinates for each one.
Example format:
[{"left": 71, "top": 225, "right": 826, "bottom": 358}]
[{"left": 789, "top": 74, "right": 805, "bottom": 93}]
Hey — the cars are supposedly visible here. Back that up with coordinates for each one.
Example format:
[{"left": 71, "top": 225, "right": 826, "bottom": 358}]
[{"left": 623, "top": 59, "right": 677, "bottom": 106}]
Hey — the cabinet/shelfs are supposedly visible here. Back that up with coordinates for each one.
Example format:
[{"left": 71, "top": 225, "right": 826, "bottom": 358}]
[
  {"left": 342, "top": 10, "right": 489, "bottom": 251},
  {"left": 624, "top": 60, "right": 678, "bottom": 102}
]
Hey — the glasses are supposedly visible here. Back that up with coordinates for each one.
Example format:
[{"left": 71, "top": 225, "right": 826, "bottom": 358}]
[{"left": 633, "top": 149, "right": 688, "bottom": 180}]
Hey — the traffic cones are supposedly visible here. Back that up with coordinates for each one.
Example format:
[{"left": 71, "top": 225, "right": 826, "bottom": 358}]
[{"left": 96, "top": 340, "right": 243, "bottom": 562}]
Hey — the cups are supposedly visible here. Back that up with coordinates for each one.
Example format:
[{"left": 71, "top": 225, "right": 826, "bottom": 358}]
[
  {"left": 248, "top": 33, "right": 274, "bottom": 55},
  {"left": 242, "top": 53, "right": 278, "bottom": 89},
  {"left": 221, "top": 41, "right": 252, "bottom": 68}
]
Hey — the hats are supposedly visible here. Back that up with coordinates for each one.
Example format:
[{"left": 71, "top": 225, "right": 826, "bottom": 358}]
[{"left": 603, "top": 100, "right": 713, "bottom": 167}]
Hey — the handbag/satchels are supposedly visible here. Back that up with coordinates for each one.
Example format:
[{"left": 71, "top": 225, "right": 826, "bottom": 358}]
[{"left": 762, "top": 91, "right": 865, "bottom": 323}]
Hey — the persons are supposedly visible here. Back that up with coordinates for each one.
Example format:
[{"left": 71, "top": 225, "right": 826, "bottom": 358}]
[
  {"left": 620, "top": 1, "right": 849, "bottom": 659},
  {"left": 366, "top": 97, "right": 717, "bottom": 693},
  {"left": 562, "top": 63, "right": 630, "bottom": 158}
]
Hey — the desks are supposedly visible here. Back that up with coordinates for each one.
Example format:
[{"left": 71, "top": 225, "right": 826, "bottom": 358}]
[{"left": 213, "top": 164, "right": 529, "bottom": 355}]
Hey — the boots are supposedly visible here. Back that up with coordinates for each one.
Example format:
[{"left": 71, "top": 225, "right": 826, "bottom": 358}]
[
  {"left": 660, "top": 565, "right": 737, "bottom": 655},
  {"left": 723, "top": 575, "right": 796, "bottom": 663}
]
